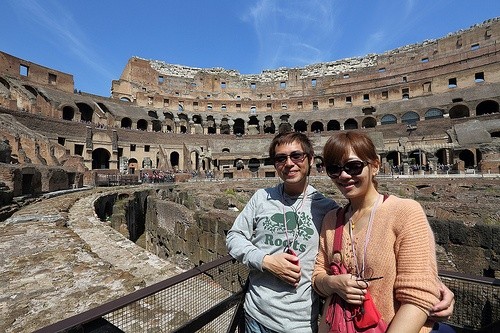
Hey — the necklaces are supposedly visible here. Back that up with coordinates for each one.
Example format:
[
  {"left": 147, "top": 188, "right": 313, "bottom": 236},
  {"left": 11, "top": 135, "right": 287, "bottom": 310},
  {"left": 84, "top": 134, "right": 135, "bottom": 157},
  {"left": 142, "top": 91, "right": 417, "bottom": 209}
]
[{"left": 352, "top": 199, "right": 376, "bottom": 229}]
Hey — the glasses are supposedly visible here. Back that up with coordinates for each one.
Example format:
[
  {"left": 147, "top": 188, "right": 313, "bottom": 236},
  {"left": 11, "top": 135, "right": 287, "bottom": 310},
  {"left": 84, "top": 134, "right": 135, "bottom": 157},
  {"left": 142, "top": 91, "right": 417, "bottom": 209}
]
[
  {"left": 273, "top": 152, "right": 308, "bottom": 166},
  {"left": 326, "top": 160, "right": 374, "bottom": 179},
  {"left": 350, "top": 266, "right": 384, "bottom": 282}
]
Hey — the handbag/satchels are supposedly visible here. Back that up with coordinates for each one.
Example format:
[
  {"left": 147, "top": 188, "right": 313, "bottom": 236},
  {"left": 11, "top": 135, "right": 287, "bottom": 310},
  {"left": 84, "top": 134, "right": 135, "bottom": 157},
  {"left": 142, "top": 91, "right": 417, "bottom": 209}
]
[{"left": 323, "top": 204, "right": 389, "bottom": 333}]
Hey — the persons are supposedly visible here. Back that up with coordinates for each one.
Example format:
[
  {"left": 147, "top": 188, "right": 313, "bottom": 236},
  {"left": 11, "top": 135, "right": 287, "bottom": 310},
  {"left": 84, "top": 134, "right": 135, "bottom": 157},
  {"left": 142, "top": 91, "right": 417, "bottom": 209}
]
[
  {"left": 71, "top": 118, "right": 217, "bottom": 137},
  {"left": 312, "top": 132, "right": 440, "bottom": 333},
  {"left": 142, "top": 170, "right": 175, "bottom": 183},
  {"left": 237, "top": 130, "right": 322, "bottom": 135},
  {"left": 225, "top": 132, "right": 455, "bottom": 333},
  {"left": 206, "top": 170, "right": 214, "bottom": 179},
  {"left": 391, "top": 161, "right": 450, "bottom": 176},
  {"left": 319, "top": 165, "right": 325, "bottom": 172},
  {"left": 192, "top": 170, "right": 197, "bottom": 178}
]
